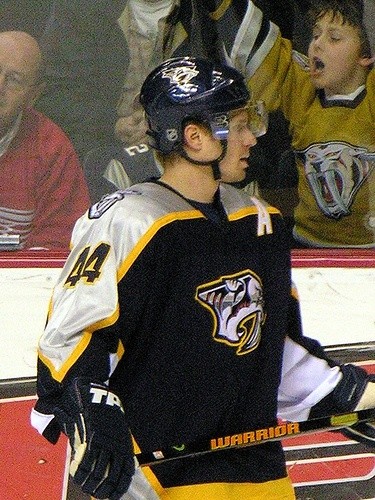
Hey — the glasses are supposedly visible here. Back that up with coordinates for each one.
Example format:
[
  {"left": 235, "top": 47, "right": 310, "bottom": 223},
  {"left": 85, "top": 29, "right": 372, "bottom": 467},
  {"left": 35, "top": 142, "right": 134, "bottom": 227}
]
[{"left": 0, "top": 64, "right": 42, "bottom": 92}]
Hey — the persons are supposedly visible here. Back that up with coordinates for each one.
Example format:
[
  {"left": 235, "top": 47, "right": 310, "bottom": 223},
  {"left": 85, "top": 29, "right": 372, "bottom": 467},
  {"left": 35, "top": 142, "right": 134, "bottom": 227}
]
[
  {"left": 30, "top": 56, "right": 375, "bottom": 500},
  {"left": 0, "top": 0, "right": 375, "bottom": 251}
]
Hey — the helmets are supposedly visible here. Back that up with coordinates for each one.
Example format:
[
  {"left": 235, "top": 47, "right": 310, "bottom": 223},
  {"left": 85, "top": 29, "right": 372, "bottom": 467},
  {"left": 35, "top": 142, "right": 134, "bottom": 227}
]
[{"left": 139, "top": 57, "right": 268, "bottom": 154}]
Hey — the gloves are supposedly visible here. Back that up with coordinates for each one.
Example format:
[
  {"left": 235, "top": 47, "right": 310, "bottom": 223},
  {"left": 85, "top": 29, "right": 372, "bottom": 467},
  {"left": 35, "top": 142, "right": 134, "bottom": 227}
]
[
  {"left": 330, "top": 363, "right": 375, "bottom": 447},
  {"left": 53, "top": 375, "right": 135, "bottom": 500}
]
